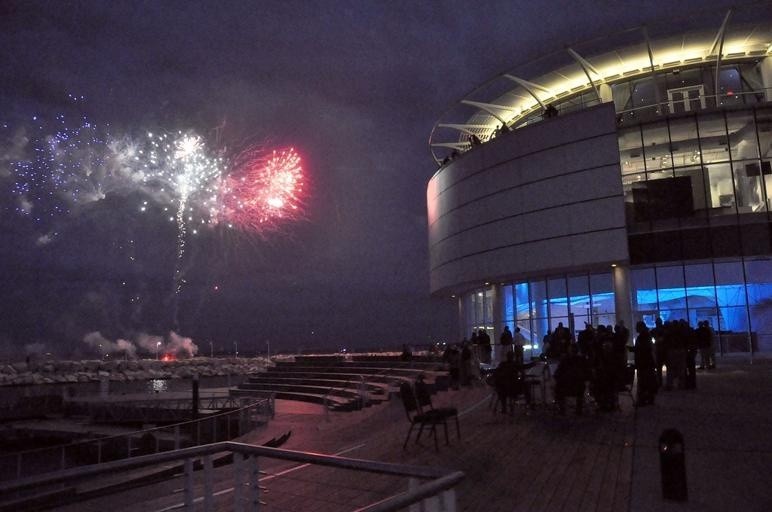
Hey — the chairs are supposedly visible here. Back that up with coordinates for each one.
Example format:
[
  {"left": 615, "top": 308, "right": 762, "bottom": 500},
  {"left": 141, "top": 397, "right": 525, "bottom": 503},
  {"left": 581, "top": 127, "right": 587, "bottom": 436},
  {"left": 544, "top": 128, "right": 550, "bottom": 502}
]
[
  {"left": 616, "top": 362, "right": 635, "bottom": 410},
  {"left": 398, "top": 374, "right": 461, "bottom": 452},
  {"left": 486, "top": 376, "right": 514, "bottom": 416}
]
